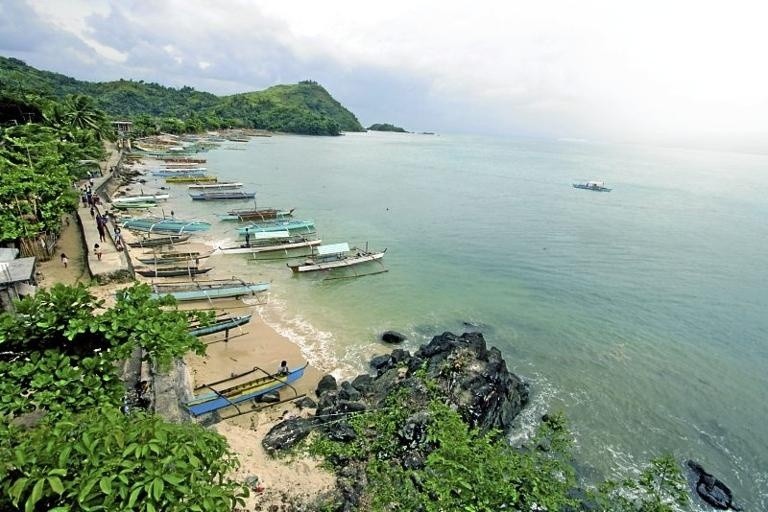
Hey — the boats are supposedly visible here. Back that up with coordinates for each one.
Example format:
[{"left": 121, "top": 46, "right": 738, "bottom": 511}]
[
  {"left": 144, "top": 276, "right": 272, "bottom": 304},
  {"left": 289, "top": 242, "right": 388, "bottom": 281},
  {"left": 176, "top": 358, "right": 309, "bottom": 426},
  {"left": 573, "top": 181, "right": 613, "bottom": 192},
  {"left": 112, "top": 126, "right": 323, "bottom": 278},
  {"left": 181, "top": 313, "right": 254, "bottom": 349}
]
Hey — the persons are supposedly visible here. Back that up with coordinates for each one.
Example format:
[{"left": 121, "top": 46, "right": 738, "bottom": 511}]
[
  {"left": 65, "top": 217, "right": 72, "bottom": 228},
  {"left": 94, "top": 244, "right": 102, "bottom": 258},
  {"left": 80, "top": 176, "right": 108, "bottom": 243},
  {"left": 114, "top": 225, "right": 122, "bottom": 247},
  {"left": 60, "top": 254, "right": 70, "bottom": 268},
  {"left": 244, "top": 228, "right": 253, "bottom": 248},
  {"left": 140, "top": 380, "right": 148, "bottom": 394},
  {"left": 277, "top": 360, "right": 289, "bottom": 377}
]
